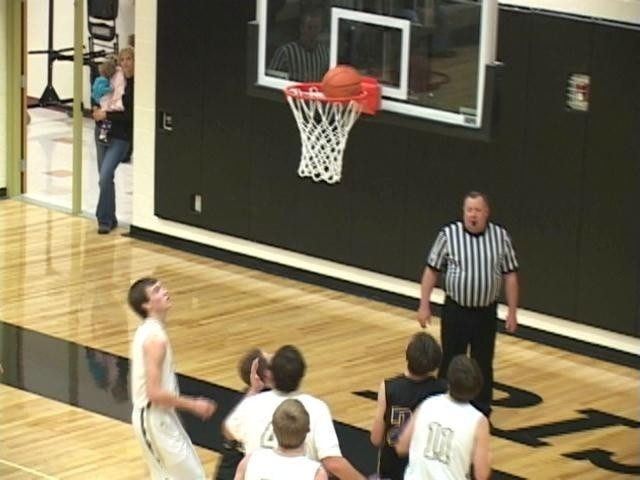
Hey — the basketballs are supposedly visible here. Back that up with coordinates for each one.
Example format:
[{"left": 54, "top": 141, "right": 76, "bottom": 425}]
[{"left": 320, "top": 66, "right": 362, "bottom": 107}]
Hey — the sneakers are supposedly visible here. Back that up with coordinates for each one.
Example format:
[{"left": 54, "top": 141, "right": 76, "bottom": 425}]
[
  {"left": 112, "top": 221, "right": 118, "bottom": 229},
  {"left": 97, "top": 221, "right": 112, "bottom": 234}
]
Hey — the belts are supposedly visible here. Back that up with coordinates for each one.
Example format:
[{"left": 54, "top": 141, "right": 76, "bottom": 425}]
[{"left": 446, "top": 295, "right": 497, "bottom": 310}]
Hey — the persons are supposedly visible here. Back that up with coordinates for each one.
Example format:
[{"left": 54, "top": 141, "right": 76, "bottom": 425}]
[
  {"left": 127, "top": 278, "right": 220, "bottom": 480},
  {"left": 214, "top": 345, "right": 279, "bottom": 480},
  {"left": 270, "top": 7, "right": 333, "bottom": 87},
  {"left": 91, "top": 59, "right": 117, "bottom": 144},
  {"left": 395, "top": 353, "right": 494, "bottom": 480},
  {"left": 234, "top": 397, "right": 330, "bottom": 480},
  {"left": 93, "top": 48, "right": 134, "bottom": 235},
  {"left": 220, "top": 342, "right": 367, "bottom": 480},
  {"left": 371, "top": 331, "right": 454, "bottom": 479},
  {"left": 415, "top": 190, "right": 519, "bottom": 425}
]
